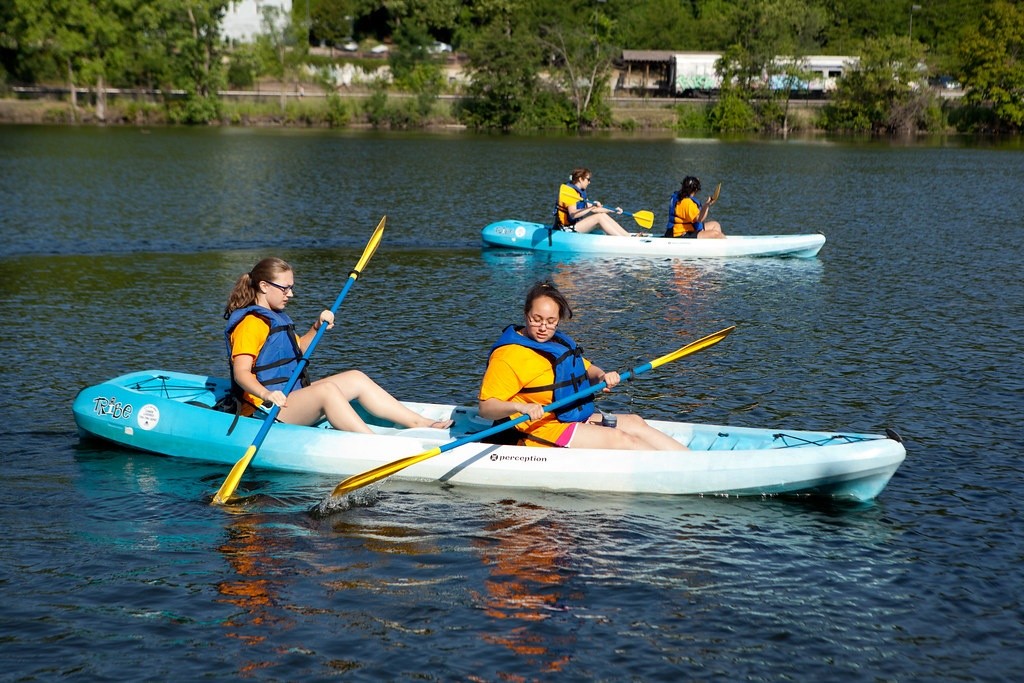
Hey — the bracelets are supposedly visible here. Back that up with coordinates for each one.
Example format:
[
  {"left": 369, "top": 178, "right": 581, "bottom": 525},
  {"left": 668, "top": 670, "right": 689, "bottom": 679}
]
[{"left": 312, "top": 324, "right": 319, "bottom": 331}]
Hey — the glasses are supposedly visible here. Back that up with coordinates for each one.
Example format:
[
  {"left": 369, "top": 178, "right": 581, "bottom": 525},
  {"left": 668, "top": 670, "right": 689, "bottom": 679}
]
[
  {"left": 257, "top": 280, "right": 295, "bottom": 294},
  {"left": 527, "top": 314, "right": 559, "bottom": 330},
  {"left": 579, "top": 176, "right": 590, "bottom": 181}
]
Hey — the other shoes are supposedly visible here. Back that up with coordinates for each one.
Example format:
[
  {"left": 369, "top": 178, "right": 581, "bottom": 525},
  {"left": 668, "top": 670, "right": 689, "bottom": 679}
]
[{"left": 635, "top": 232, "right": 642, "bottom": 237}]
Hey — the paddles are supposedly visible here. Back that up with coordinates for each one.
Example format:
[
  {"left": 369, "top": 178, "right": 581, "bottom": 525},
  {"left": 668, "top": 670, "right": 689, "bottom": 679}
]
[
  {"left": 558, "top": 183, "right": 655, "bottom": 230},
  {"left": 330, "top": 322, "right": 738, "bottom": 499},
  {"left": 703, "top": 182, "right": 722, "bottom": 207},
  {"left": 209, "top": 212, "right": 390, "bottom": 509}
]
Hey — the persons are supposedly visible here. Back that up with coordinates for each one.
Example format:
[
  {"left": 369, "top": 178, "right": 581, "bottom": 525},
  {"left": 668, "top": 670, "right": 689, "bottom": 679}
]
[
  {"left": 554, "top": 167, "right": 643, "bottom": 236},
  {"left": 476, "top": 278, "right": 695, "bottom": 452},
  {"left": 664, "top": 176, "right": 727, "bottom": 238},
  {"left": 222, "top": 257, "right": 454, "bottom": 436}
]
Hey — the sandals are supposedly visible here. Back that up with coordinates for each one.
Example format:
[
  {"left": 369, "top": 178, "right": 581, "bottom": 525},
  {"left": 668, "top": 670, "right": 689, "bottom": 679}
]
[{"left": 428, "top": 418, "right": 453, "bottom": 430}]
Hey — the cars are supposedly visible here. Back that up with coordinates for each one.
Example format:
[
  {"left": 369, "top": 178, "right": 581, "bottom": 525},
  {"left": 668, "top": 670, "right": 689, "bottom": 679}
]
[
  {"left": 430, "top": 41, "right": 452, "bottom": 55},
  {"left": 333, "top": 37, "right": 359, "bottom": 52},
  {"left": 931, "top": 74, "right": 964, "bottom": 91}
]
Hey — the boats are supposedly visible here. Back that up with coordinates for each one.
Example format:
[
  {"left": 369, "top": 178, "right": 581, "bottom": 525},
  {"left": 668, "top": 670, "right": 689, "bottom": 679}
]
[
  {"left": 480, "top": 218, "right": 827, "bottom": 260},
  {"left": 73, "top": 369, "right": 907, "bottom": 508}
]
